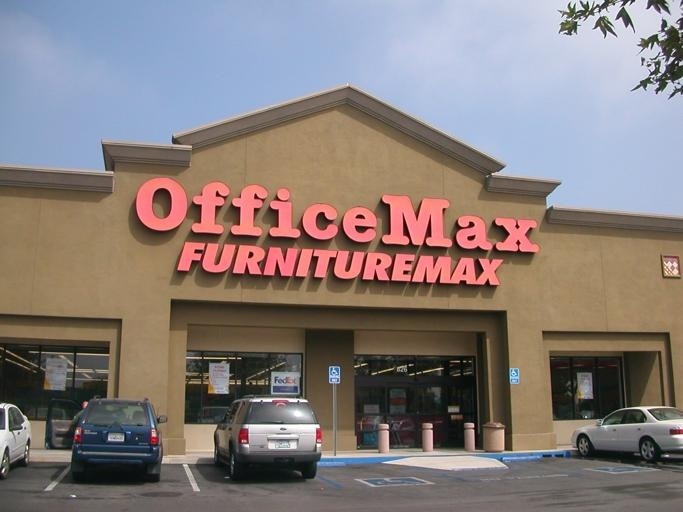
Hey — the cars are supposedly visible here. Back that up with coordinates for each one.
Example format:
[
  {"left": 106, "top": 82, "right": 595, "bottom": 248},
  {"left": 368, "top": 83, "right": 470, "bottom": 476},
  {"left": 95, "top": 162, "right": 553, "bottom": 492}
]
[
  {"left": 197, "top": 406, "right": 229, "bottom": 423},
  {"left": 214, "top": 396, "right": 322, "bottom": 480},
  {"left": 0, "top": 403, "right": 31, "bottom": 478},
  {"left": 45, "top": 400, "right": 167, "bottom": 481},
  {"left": 27, "top": 407, "right": 67, "bottom": 420},
  {"left": 570, "top": 405, "right": 683, "bottom": 461}
]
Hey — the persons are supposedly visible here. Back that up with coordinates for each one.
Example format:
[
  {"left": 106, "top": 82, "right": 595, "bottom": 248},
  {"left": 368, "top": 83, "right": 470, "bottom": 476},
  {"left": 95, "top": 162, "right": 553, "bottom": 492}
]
[{"left": 70, "top": 401, "right": 88, "bottom": 436}]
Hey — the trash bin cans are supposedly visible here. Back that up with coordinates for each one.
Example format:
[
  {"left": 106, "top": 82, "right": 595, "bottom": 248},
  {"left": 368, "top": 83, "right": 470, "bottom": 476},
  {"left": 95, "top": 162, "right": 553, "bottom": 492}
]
[{"left": 482, "top": 422, "right": 506, "bottom": 452}]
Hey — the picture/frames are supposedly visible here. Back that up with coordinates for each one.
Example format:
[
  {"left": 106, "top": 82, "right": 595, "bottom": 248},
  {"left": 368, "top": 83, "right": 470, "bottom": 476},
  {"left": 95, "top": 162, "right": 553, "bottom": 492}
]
[{"left": 661, "top": 254, "right": 681, "bottom": 279}]
